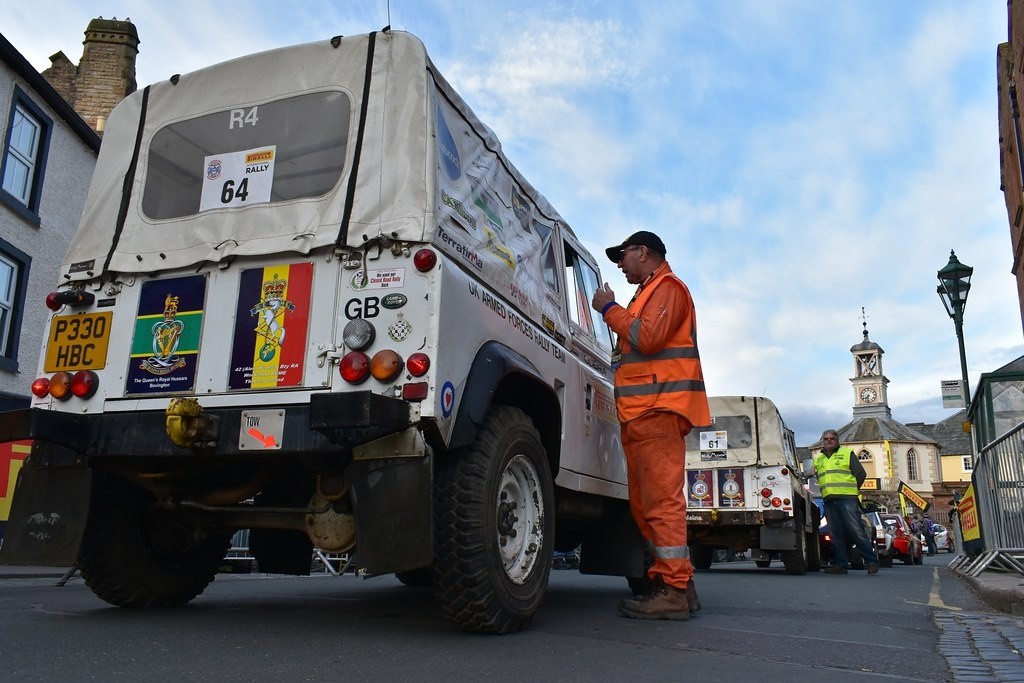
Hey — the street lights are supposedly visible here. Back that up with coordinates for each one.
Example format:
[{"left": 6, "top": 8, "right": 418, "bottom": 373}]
[{"left": 935, "top": 250, "right": 975, "bottom": 472}]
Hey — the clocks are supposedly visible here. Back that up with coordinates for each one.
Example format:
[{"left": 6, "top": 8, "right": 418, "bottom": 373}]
[{"left": 860, "top": 388, "right": 876, "bottom": 403}]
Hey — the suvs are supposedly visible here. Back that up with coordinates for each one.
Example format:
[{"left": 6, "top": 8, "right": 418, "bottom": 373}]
[
  {"left": 26, "top": 32, "right": 664, "bottom": 633},
  {"left": 678, "top": 395, "right": 820, "bottom": 574}
]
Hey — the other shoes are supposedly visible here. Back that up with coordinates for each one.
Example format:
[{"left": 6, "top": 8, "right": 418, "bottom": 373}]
[
  {"left": 868, "top": 563, "right": 878, "bottom": 575},
  {"left": 825, "top": 566, "right": 848, "bottom": 574},
  {"left": 927, "top": 553, "right": 933, "bottom": 556}
]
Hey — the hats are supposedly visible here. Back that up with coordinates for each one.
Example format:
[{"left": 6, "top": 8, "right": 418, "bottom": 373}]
[{"left": 606, "top": 232, "right": 666, "bottom": 264}]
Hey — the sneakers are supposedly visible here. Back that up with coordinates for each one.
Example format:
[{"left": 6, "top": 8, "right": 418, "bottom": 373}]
[
  {"left": 685, "top": 580, "right": 701, "bottom": 612},
  {"left": 619, "top": 583, "right": 689, "bottom": 620}
]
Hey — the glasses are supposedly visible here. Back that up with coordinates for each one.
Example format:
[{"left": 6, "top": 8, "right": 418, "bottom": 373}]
[
  {"left": 618, "top": 248, "right": 640, "bottom": 262},
  {"left": 823, "top": 437, "right": 836, "bottom": 441}
]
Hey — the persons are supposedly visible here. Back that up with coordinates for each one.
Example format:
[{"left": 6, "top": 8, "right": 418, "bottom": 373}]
[
  {"left": 592, "top": 230, "right": 711, "bottom": 621},
  {"left": 813, "top": 429, "right": 878, "bottom": 575},
  {"left": 911, "top": 500, "right": 960, "bottom": 556}
]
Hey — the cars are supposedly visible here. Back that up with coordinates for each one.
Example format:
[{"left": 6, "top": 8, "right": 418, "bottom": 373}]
[
  {"left": 879, "top": 513, "right": 923, "bottom": 565},
  {"left": 818, "top": 511, "right": 894, "bottom": 569},
  {"left": 920, "top": 524, "right": 955, "bottom": 553}
]
